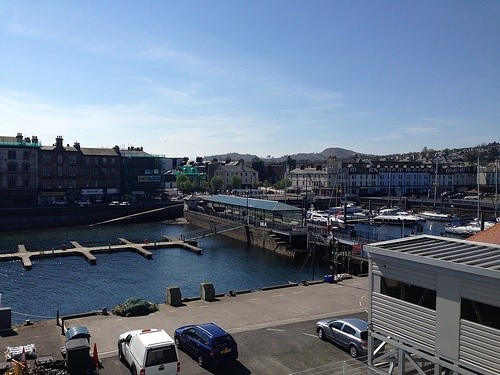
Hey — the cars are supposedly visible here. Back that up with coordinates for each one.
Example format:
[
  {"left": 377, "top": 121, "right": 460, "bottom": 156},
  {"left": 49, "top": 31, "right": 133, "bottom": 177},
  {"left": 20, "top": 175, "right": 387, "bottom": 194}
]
[
  {"left": 74, "top": 200, "right": 92, "bottom": 206},
  {"left": 174, "top": 323, "right": 238, "bottom": 367},
  {"left": 315, "top": 317, "right": 385, "bottom": 358},
  {"left": 118, "top": 326, "right": 181, "bottom": 375},
  {"left": 50, "top": 198, "right": 68, "bottom": 206},
  {"left": 109, "top": 201, "right": 129, "bottom": 205}
]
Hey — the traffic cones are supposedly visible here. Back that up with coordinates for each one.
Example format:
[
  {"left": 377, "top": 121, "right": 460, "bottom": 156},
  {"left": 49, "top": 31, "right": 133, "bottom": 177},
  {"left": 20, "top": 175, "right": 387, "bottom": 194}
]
[
  {"left": 92, "top": 343, "right": 100, "bottom": 368},
  {"left": 21, "top": 347, "right": 27, "bottom": 368}
]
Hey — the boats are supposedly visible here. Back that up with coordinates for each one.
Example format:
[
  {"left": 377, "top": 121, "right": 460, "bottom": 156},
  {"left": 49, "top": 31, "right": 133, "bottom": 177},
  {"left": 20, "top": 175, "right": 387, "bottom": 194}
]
[
  {"left": 372, "top": 208, "right": 426, "bottom": 225},
  {"left": 418, "top": 211, "right": 455, "bottom": 222},
  {"left": 329, "top": 209, "right": 378, "bottom": 220},
  {"left": 444, "top": 218, "right": 496, "bottom": 235},
  {"left": 305, "top": 210, "right": 344, "bottom": 225},
  {"left": 329, "top": 201, "right": 361, "bottom": 213}
]
